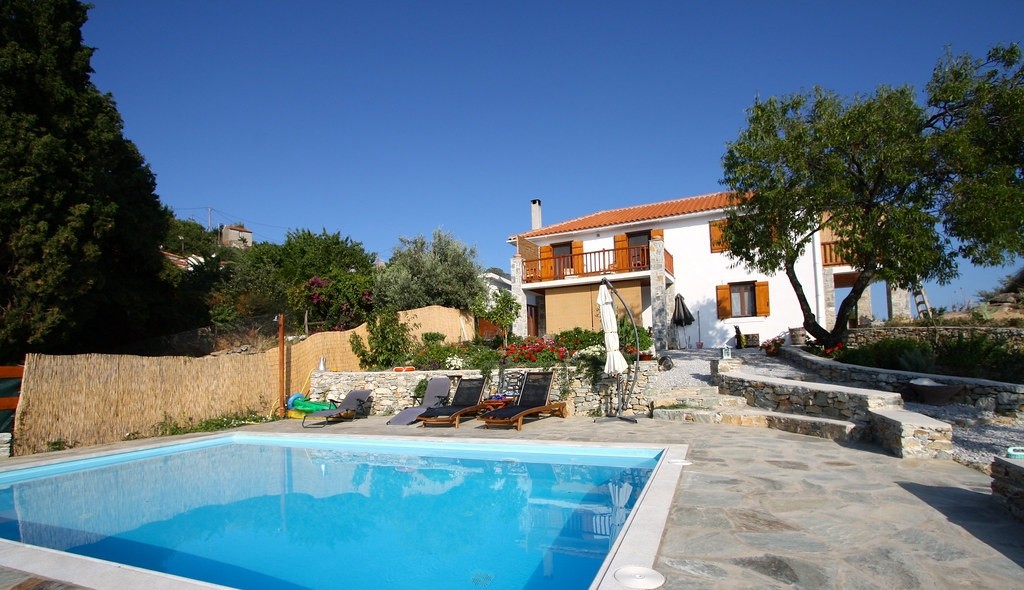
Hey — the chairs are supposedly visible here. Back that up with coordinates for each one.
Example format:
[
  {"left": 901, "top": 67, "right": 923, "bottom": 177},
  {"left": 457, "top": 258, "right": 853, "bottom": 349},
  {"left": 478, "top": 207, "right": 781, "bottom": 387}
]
[
  {"left": 386, "top": 377, "right": 488, "bottom": 430},
  {"left": 302, "top": 389, "right": 373, "bottom": 429},
  {"left": 476, "top": 372, "right": 567, "bottom": 431}
]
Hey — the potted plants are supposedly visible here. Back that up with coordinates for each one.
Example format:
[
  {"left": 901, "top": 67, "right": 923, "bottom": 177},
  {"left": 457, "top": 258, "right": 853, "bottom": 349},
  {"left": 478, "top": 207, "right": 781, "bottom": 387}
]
[
  {"left": 759, "top": 335, "right": 786, "bottom": 357},
  {"left": 639, "top": 352, "right": 651, "bottom": 362}
]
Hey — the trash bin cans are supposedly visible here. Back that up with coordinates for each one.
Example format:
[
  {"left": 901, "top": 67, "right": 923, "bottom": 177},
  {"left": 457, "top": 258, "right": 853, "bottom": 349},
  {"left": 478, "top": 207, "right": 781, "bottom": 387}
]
[{"left": 788, "top": 325, "right": 807, "bottom": 346}]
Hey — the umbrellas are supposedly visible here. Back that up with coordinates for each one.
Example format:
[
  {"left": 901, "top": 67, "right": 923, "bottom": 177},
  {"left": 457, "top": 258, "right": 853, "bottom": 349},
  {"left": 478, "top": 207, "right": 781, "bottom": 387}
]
[
  {"left": 596, "top": 284, "right": 628, "bottom": 416},
  {"left": 670, "top": 293, "right": 695, "bottom": 349}
]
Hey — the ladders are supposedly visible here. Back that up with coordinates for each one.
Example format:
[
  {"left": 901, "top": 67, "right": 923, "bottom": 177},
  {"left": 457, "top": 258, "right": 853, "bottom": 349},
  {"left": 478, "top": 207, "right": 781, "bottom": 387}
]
[{"left": 912, "top": 274, "right": 935, "bottom": 321}]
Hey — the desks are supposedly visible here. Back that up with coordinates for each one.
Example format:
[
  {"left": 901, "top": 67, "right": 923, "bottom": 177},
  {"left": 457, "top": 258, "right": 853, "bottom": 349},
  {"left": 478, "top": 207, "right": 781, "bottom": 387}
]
[{"left": 483, "top": 396, "right": 512, "bottom": 420}]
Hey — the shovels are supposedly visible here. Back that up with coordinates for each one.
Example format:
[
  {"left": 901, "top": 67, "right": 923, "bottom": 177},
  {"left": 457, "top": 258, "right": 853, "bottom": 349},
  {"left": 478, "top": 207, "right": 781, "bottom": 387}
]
[{"left": 694, "top": 310, "right": 703, "bottom": 349}]
